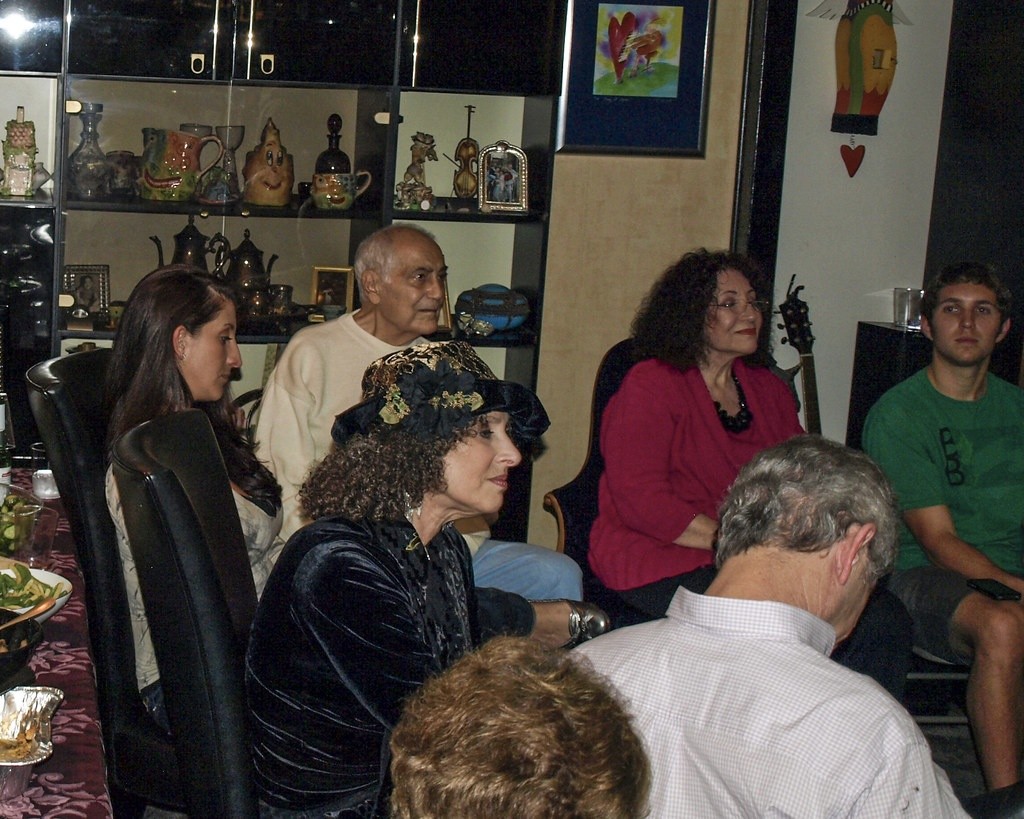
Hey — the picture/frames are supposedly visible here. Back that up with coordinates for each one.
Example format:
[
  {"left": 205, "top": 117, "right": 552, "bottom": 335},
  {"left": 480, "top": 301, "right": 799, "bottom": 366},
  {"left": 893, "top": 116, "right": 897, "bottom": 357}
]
[
  {"left": 554, "top": 0, "right": 718, "bottom": 161},
  {"left": 478, "top": 140, "right": 529, "bottom": 212},
  {"left": 64, "top": 264, "right": 110, "bottom": 311},
  {"left": 311, "top": 264, "right": 354, "bottom": 318}
]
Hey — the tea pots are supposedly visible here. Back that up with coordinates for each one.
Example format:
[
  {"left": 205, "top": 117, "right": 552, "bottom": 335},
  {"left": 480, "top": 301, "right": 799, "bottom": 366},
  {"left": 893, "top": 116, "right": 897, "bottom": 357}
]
[
  {"left": 149, "top": 214, "right": 231, "bottom": 276},
  {"left": 216, "top": 228, "right": 280, "bottom": 290}
]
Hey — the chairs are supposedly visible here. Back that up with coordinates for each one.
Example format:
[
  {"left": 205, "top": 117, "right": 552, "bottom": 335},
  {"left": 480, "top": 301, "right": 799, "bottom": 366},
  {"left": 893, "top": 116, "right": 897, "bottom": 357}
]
[
  {"left": 540, "top": 338, "right": 967, "bottom": 730},
  {"left": 20, "top": 348, "right": 266, "bottom": 819}
]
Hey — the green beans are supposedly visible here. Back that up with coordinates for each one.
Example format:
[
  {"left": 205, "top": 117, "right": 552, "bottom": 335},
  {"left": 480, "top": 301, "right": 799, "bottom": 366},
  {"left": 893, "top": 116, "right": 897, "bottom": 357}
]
[{"left": 0, "top": 563, "right": 67, "bottom": 610}]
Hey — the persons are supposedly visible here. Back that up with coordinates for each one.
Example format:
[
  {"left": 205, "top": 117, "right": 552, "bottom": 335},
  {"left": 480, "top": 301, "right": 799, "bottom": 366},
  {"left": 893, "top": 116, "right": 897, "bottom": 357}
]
[
  {"left": 104, "top": 266, "right": 610, "bottom": 734},
  {"left": 487, "top": 162, "right": 519, "bottom": 203},
  {"left": 240, "top": 340, "right": 521, "bottom": 818},
  {"left": 566, "top": 435, "right": 968, "bottom": 819},
  {"left": 75, "top": 275, "right": 101, "bottom": 313},
  {"left": 389, "top": 629, "right": 654, "bottom": 819},
  {"left": 588, "top": 247, "right": 810, "bottom": 617},
  {"left": 861, "top": 264, "right": 1024, "bottom": 818},
  {"left": 253, "top": 224, "right": 584, "bottom": 612}
]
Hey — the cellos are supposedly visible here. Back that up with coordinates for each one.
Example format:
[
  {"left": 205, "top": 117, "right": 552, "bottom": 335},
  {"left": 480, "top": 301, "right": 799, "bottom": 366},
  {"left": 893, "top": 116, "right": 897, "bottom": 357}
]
[{"left": 453, "top": 105, "right": 479, "bottom": 198}]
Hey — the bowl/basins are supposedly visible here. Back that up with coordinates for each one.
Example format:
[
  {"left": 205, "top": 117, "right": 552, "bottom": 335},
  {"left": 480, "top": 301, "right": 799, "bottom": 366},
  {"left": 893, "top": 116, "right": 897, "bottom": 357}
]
[
  {"left": 239, "top": 305, "right": 320, "bottom": 335},
  {"left": 0, "top": 569, "right": 73, "bottom": 624},
  {"left": 0, "top": 607, "right": 43, "bottom": 684},
  {"left": 0, "top": 483, "right": 44, "bottom": 560}
]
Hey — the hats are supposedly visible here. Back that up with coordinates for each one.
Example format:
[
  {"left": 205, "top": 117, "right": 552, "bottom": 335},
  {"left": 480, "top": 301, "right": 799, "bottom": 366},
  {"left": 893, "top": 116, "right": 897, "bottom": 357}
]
[{"left": 330, "top": 339, "right": 551, "bottom": 448}]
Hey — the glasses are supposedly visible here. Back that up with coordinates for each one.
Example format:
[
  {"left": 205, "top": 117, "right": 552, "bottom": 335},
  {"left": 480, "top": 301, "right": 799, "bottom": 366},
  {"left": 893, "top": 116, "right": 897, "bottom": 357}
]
[{"left": 710, "top": 296, "right": 770, "bottom": 315}]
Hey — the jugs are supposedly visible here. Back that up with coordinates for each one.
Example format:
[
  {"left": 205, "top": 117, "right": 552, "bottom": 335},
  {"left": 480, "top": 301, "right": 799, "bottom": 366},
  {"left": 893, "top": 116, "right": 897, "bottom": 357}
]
[{"left": 142, "top": 127, "right": 223, "bottom": 202}]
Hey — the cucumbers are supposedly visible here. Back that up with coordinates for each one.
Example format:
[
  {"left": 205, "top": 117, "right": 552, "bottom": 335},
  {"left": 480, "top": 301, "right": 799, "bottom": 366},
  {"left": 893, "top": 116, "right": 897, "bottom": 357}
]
[{"left": 0, "top": 494, "right": 34, "bottom": 554}]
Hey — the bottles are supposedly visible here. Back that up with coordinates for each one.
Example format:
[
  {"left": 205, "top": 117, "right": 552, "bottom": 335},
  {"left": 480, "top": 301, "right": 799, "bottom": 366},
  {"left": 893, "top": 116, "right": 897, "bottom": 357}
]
[
  {"left": 0, "top": 400, "right": 12, "bottom": 507},
  {"left": 67, "top": 101, "right": 112, "bottom": 196},
  {"left": 315, "top": 113, "right": 351, "bottom": 174}
]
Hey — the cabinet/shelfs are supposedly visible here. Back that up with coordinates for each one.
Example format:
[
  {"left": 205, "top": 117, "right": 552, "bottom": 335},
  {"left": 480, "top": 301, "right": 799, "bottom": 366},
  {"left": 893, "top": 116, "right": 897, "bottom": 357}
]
[{"left": 1, "top": 0, "right": 568, "bottom": 548}]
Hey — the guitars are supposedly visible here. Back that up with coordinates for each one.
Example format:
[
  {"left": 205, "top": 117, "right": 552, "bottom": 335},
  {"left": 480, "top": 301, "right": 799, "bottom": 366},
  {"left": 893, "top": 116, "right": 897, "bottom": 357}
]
[{"left": 773, "top": 293, "right": 822, "bottom": 435}]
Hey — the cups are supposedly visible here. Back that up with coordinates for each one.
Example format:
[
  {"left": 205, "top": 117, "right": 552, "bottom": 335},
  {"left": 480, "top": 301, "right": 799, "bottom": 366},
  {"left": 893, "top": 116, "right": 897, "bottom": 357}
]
[
  {"left": 107, "top": 151, "right": 146, "bottom": 197},
  {"left": 893, "top": 288, "right": 922, "bottom": 326},
  {"left": 310, "top": 171, "right": 372, "bottom": 209},
  {"left": 14, "top": 505, "right": 59, "bottom": 569},
  {"left": 228, "top": 284, "right": 294, "bottom": 315},
  {"left": 31, "top": 443, "right": 60, "bottom": 499},
  {"left": 0, "top": 393, "right": 15, "bottom": 450}
]
